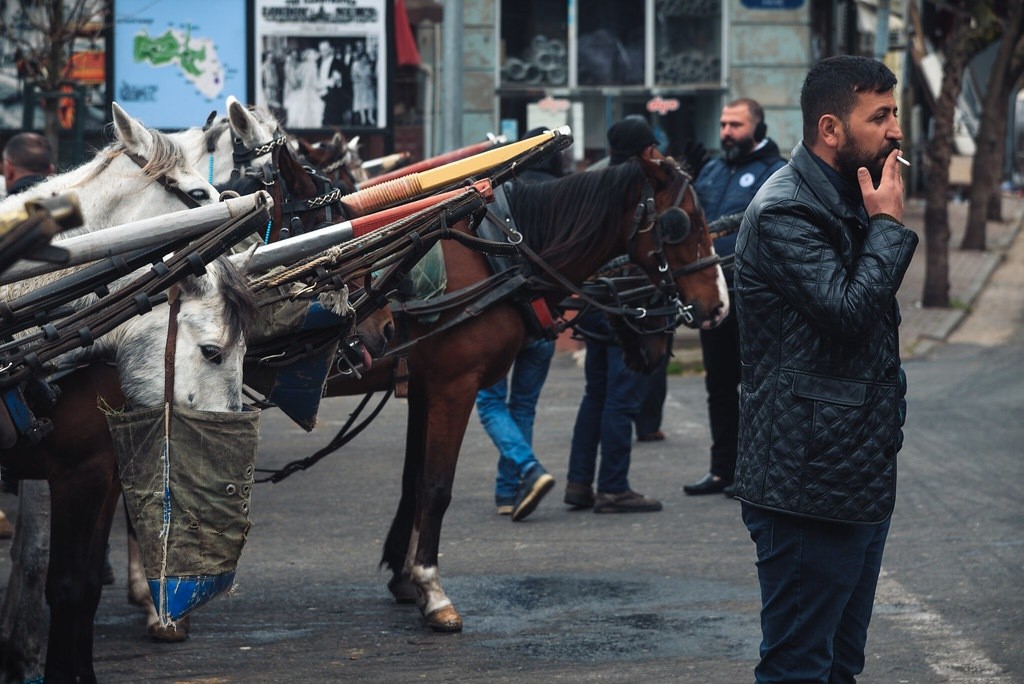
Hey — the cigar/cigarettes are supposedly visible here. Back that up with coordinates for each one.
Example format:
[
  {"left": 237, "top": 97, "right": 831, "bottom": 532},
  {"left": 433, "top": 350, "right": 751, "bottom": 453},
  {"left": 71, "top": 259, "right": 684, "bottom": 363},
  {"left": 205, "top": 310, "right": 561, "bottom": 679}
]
[{"left": 896, "top": 156, "right": 910, "bottom": 167}]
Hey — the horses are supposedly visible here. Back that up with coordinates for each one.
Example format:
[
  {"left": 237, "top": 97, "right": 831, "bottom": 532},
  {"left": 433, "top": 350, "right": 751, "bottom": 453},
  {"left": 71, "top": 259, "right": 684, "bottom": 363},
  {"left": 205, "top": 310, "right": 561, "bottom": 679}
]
[{"left": 3, "top": 93, "right": 729, "bottom": 684}]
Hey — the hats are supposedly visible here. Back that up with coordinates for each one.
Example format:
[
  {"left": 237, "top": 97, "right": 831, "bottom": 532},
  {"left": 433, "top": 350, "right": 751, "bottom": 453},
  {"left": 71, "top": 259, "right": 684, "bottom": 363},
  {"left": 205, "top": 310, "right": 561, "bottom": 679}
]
[{"left": 609, "top": 118, "right": 661, "bottom": 158}]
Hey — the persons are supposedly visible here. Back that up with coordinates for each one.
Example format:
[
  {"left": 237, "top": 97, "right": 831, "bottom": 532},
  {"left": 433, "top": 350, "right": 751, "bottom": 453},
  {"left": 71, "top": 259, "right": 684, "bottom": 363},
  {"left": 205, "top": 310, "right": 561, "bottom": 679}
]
[
  {"left": 633, "top": 357, "right": 670, "bottom": 441},
  {"left": 1, "top": 131, "right": 61, "bottom": 196},
  {"left": 731, "top": 56, "right": 919, "bottom": 684},
  {"left": 475, "top": 127, "right": 555, "bottom": 523},
  {"left": 561, "top": 116, "right": 667, "bottom": 515},
  {"left": 259, "top": 40, "right": 380, "bottom": 130},
  {"left": 683, "top": 97, "right": 790, "bottom": 497}
]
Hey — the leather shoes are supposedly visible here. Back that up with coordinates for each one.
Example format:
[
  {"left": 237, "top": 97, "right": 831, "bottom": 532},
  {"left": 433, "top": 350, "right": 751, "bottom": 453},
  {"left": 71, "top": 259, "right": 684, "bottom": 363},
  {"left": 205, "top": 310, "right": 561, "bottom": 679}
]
[{"left": 684, "top": 473, "right": 727, "bottom": 495}]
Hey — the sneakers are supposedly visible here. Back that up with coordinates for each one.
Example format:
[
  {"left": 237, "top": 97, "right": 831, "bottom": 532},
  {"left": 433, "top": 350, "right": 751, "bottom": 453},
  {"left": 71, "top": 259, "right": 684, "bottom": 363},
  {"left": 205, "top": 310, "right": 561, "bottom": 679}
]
[
  {"left": 595, "top": 488, "right": 660, "bottom": 515},
  {"left": 565, "top": 483, "right": 596, "bottom": 508},
  {"left": 497, "top": 464, "right": 553, "bottom": 522}
]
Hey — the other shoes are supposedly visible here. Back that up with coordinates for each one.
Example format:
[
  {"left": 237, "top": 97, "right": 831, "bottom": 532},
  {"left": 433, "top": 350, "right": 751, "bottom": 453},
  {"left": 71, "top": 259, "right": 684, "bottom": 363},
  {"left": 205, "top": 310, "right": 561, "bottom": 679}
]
[{"left": 640, "top": 430, "right": 665, "bottom": 439}]
